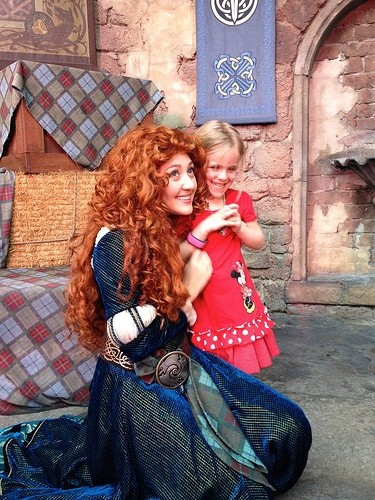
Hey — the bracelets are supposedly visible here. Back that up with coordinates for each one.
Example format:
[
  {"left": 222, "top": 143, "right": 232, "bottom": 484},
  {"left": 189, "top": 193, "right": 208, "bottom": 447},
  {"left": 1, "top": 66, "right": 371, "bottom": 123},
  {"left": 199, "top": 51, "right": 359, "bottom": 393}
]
[{"left": 186, "top": 232, "right": 208, "bottom": 250}]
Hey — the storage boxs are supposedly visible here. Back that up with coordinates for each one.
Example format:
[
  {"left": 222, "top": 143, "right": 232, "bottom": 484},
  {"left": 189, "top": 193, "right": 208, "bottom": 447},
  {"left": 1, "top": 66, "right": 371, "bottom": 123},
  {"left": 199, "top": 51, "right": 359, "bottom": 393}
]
[{"left": 0, "top": 61, "right": 164, "bottom": 173}]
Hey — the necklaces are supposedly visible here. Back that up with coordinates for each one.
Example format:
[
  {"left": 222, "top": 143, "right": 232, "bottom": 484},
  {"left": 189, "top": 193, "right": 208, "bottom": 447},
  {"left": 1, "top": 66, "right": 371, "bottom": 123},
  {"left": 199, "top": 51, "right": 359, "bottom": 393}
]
[{"left": 206, "top": 195, "right": 228, "bottom": 236}]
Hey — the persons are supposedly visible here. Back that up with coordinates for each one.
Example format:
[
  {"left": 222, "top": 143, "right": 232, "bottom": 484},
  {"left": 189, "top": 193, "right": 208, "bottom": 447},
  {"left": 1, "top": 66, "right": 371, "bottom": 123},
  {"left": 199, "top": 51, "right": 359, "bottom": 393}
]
[
  {"left": 1, "top": 124, "right": 313, "bottom": 500},
  {"left": 173, "top": 120, "right": 280, "bottom": 374}
]
